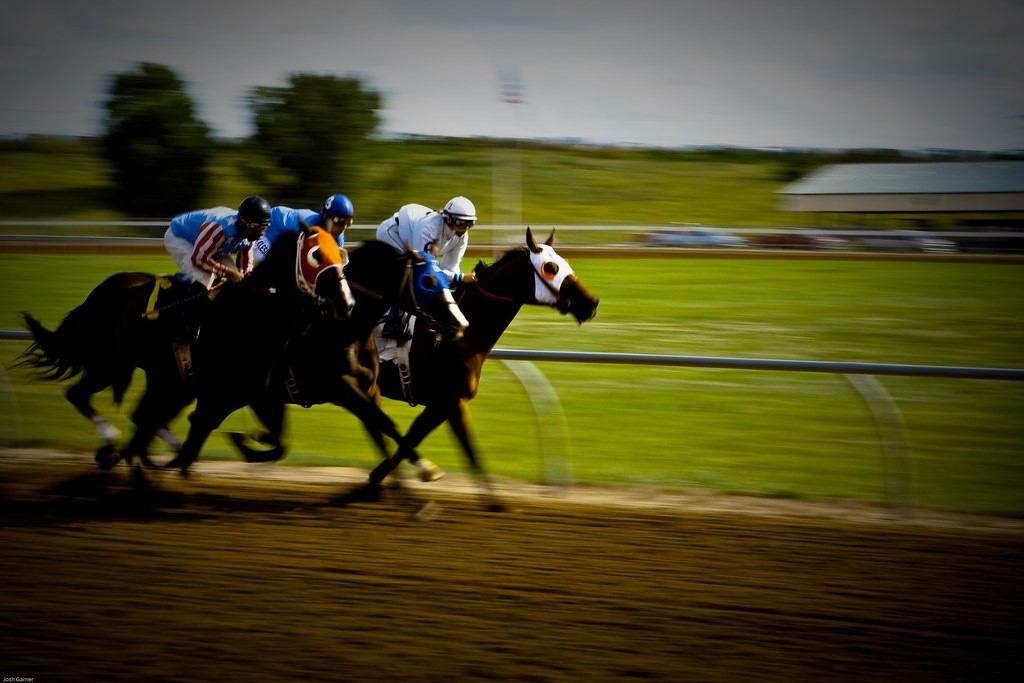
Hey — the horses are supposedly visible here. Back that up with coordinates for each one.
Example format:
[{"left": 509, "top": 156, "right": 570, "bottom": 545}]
[{"left": 5, "top": 218, "right": 602, "bottom": 513}]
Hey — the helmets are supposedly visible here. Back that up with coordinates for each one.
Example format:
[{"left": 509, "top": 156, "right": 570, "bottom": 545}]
[
  {"left": 323, "top": 194, "right": 354, "bottom": 218},
  {"left": 441, "top": 196, "right": 477, "bottom": 220},
  {"left": 238, "top": 197, "right": 271, "bottom": 221}
]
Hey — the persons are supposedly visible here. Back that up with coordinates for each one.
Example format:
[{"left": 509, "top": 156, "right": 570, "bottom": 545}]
[
  {"left": 162, "top": 196, "right": 274, "bottom": 344},
  {"left": 375, "top": 195, "right": 478, "bottom": 340},
  {"left": 252, "top": 194, "right": 355, "bottom": 274}
]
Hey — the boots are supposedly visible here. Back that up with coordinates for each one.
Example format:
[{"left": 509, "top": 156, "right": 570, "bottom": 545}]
[
  {"left": 382, "top": 307, "right": 412, "bottom": 341},
  {"left": 168, "top": 281, "right": 208, "bottom": 342}
]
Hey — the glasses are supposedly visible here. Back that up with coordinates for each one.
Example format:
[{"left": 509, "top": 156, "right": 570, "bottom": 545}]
[
  {"left": 239, "top": 218, "right": 270, "bottom": 230},
  {"left": 444, "top": 211, "right": 474, "bottom": 228},
  {"left": 322, "top": 207, "right": 353, "bottom": 226}
]
